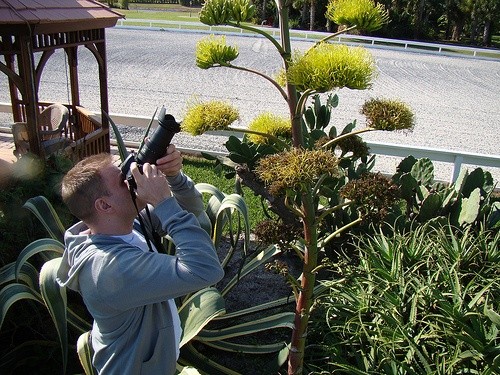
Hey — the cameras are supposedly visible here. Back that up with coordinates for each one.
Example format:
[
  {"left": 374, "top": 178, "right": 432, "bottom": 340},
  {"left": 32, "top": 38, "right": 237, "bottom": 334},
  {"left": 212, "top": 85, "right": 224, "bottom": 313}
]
[{"left": 120, "top": 106, "right": 182, "bottom": 190}]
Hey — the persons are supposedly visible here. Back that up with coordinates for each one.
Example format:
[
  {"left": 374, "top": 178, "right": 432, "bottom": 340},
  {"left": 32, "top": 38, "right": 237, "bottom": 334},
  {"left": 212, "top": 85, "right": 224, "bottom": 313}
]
[{"left": 55, "top": 141, "right": 226, "bottom": 375}]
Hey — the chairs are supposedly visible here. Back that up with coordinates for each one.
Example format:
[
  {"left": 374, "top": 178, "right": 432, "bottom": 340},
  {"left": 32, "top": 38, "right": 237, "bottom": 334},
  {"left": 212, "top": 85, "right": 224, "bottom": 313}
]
[{"left": 10, "top": 102, "right": 69, "bottom": 160}]
